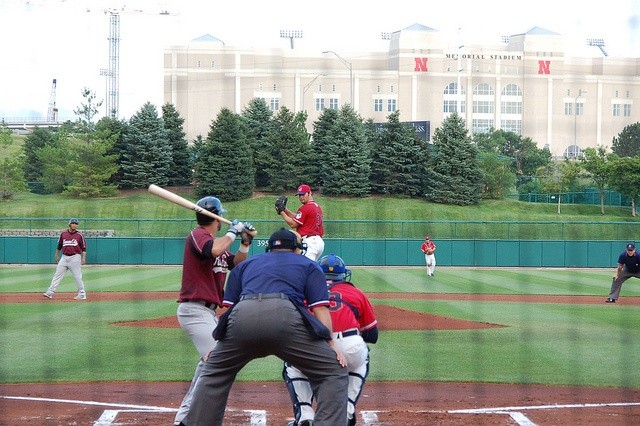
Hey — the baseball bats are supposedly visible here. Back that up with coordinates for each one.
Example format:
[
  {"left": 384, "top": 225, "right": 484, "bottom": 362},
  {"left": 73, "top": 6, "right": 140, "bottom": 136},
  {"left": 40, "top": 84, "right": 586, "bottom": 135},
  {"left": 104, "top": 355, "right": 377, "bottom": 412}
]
[{"left": 148, "top": 184, "right": 257, "bottom": 237}]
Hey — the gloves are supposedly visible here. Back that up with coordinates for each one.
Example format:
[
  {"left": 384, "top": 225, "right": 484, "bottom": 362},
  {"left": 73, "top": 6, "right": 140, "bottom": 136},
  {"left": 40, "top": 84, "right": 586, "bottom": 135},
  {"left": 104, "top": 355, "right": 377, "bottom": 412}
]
[
  {"left": 274, "top": 200, "right": 285, "bottom": 215},
  {"left": 279, "top": 195, "right": 287, "bottom": 206},
  {"left": 240, "top": 222, "right": 255, "bottom": 245},
  {"left": 227, "top": 219, "right": 243, "bottom": 234}
]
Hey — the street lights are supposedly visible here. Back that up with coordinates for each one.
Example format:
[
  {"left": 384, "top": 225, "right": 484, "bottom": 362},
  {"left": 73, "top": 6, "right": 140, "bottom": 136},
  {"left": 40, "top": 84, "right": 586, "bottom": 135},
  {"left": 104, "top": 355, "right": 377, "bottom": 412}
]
[
  {"left": 572, "top": 88, "right": 589, "bottom": 162},
  {"left": 297, "top": 71, "right": 329, "bottom": 120},
  {"left": 321, "top": 49, "right": 355, "bottom": 111}
]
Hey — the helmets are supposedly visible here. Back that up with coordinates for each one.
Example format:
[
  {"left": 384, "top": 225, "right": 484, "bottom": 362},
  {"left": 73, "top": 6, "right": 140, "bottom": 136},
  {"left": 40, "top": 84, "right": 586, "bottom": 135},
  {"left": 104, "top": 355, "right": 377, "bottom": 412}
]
[
  {"left": 196, "top": 196, "right": 228, "bottom": 222},
  {"left": 69, "top": 218, "right": 78, "bottom": 225},
  {"left": 266, "top": 228, "right": 307, "bottom": 256},
  {"left": 316, "top": 254, "right": 351, "bottom": 283}
]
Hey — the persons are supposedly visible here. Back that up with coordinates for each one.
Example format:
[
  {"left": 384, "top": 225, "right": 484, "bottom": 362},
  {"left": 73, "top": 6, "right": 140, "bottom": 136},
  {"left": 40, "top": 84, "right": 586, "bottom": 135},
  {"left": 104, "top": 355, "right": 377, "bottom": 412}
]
[
  {"left": 275, "top": 184, "right": 324, "bottom": 263},
  {"left": 179, "top": 228, "right": 349, "bottom": 426},
  {"left": 282, "top": 253, "right": 378, "bottom": 426},
  {"left": 605, "top": 244, "right": 640, "bottom": 302},
  {"left": 42, "top": 217, "right": 87, "bottom": 301},
  {"left": 173, "top": 196, "right": 255, "bottom": 426},
  {"left": 421, "top": 237, "right": 437, "bottom": 277}
]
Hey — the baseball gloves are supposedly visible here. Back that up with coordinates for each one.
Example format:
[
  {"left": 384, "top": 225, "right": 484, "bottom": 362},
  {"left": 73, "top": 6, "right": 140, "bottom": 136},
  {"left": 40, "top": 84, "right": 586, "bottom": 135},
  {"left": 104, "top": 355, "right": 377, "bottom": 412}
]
[{"left": 275, "top": 196, "right": 288, "bottom": 215}]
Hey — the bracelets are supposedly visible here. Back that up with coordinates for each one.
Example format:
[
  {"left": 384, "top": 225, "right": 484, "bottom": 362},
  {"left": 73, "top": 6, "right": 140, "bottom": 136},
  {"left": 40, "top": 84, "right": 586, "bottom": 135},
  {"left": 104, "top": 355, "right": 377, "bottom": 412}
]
[
  {"left": 239, "top": 244, "right": 249, "bottom": 253},
  {"left": 225, "top": 231, "right": 237, "bottom": 242}
]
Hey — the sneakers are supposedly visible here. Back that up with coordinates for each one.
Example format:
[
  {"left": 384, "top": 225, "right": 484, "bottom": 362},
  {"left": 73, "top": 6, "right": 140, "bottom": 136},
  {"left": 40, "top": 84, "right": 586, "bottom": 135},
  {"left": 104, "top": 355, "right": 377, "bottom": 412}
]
[
  {"left": 43, "top": 293, "right": 52, "bottom": 299},
  {"left": 606, "top": 298, "right": 615, "bottom": 302},
  {"left": 73, "top": 295, "right": 86, "bottom": 300}
]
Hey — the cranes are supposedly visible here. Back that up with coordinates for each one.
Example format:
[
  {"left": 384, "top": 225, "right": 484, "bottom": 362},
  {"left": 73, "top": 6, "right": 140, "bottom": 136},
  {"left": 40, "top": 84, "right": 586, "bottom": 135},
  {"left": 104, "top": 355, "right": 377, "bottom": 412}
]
[{"left": 17, "top": 0, "right": 181, "bottom": 121}]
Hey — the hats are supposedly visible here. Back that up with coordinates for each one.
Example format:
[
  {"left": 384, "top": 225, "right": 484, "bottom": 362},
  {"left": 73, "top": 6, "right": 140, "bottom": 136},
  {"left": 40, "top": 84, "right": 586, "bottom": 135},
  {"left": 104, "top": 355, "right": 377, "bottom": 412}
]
[
  {"left": 426, "top": 236, "right": 430, "bottom": 240},
  {"left": 627, "top": 244, "right": 635, "bottom": 251},
  {"left": 295, "top": 185, "right": 312, "bottom": 196}
]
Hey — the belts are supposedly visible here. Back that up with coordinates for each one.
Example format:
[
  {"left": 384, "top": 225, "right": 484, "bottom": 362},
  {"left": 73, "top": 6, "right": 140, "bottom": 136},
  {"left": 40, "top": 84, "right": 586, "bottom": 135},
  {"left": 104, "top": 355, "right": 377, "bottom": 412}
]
[
  {"left": 337, "top": 330, "right": 357, "bottom": 338},
  {"left": 182, "top": 299, "right": 217, "bottom": 310},
  {"left": 240, "top": 292, "right": 287, "bottom": 299}
]
